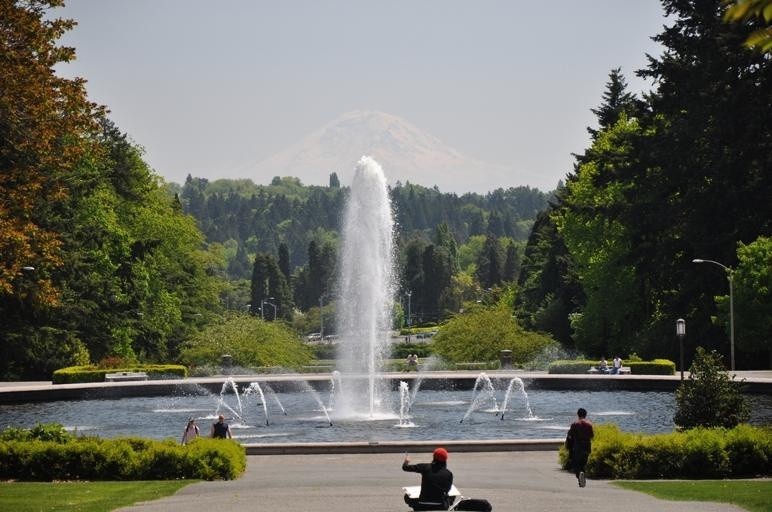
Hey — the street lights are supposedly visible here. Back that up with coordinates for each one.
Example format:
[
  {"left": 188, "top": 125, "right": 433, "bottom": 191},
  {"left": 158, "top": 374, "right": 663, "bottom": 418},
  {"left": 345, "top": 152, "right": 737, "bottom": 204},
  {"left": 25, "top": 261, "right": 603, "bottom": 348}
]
[
  {"left": 318, "top": 296, "right": 324, "bottom": 336},
  {"left": 675, "top": 318, "right": 686, "bottom": 381},
  {"left": 240, "top": 297, "right": 277, "bottom": 322},
  {"left": 694, "top": 259, "right": 735, "bottom": 371},
  {"left": 407, "top": 293, "right": 412, "bottom": 329}
]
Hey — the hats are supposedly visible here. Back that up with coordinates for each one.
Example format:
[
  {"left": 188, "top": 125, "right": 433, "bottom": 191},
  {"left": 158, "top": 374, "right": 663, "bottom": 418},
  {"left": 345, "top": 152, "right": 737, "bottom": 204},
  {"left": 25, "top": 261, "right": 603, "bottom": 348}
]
[{"left": 433, "top": 448, "right": 449, "bottom": 461}]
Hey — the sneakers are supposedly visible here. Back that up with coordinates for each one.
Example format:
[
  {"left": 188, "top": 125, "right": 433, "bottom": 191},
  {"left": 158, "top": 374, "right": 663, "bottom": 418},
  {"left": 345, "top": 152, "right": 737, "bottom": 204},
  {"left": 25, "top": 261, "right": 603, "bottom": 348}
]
[{"left": 576, "top": 472, "right": 586, "bottom": 487}]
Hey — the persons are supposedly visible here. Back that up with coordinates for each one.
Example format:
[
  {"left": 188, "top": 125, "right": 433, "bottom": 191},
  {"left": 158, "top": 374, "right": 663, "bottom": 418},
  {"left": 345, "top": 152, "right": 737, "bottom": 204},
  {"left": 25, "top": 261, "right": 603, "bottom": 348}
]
[
  {"left": 609, "top": 355, "right": 622, "bottom": 374},
  {"left": 402, "top": 447, "right": 453, "bottom": 512},
  {"left": 210, "top": 415, "right": 232, "bottom": 439},
  {"left": 180, "top": 416, "right": 200, "bottom": 447},
  {"left": 599, "top": 356, "right": 607, "bottom": 374},
  {"left": 565, "top": 408, "right": 594, "bottom": 487},
  {"left": 406, "top": 352, "right": 419, "bottom": 373}
]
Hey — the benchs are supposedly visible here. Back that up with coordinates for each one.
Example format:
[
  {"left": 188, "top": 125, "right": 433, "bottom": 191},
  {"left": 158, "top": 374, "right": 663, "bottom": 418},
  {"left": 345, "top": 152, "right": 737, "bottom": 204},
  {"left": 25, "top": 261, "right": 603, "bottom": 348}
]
[
  {"left": 105, "top": 371, "right": 150, "bottom": 382},
  {"left": 587, "top": 366, "right": 631, "bottom": 375},
  {"left": 400, "top": 363, "right": 432, "bottom": 374}
]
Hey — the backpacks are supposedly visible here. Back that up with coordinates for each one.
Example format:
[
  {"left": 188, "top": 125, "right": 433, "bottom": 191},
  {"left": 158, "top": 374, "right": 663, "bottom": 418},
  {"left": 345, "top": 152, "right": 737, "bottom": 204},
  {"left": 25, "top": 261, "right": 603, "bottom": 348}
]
[{"left": 454, "top": 498, "right": 492, "bottom": 512}]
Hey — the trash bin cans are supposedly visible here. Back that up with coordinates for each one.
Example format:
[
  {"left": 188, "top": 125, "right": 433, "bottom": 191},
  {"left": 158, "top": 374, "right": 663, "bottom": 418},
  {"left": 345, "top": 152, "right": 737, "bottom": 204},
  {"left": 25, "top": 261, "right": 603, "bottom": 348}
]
[{"left": 500, "top": 350, "right": 512, "bottom": 369}]
